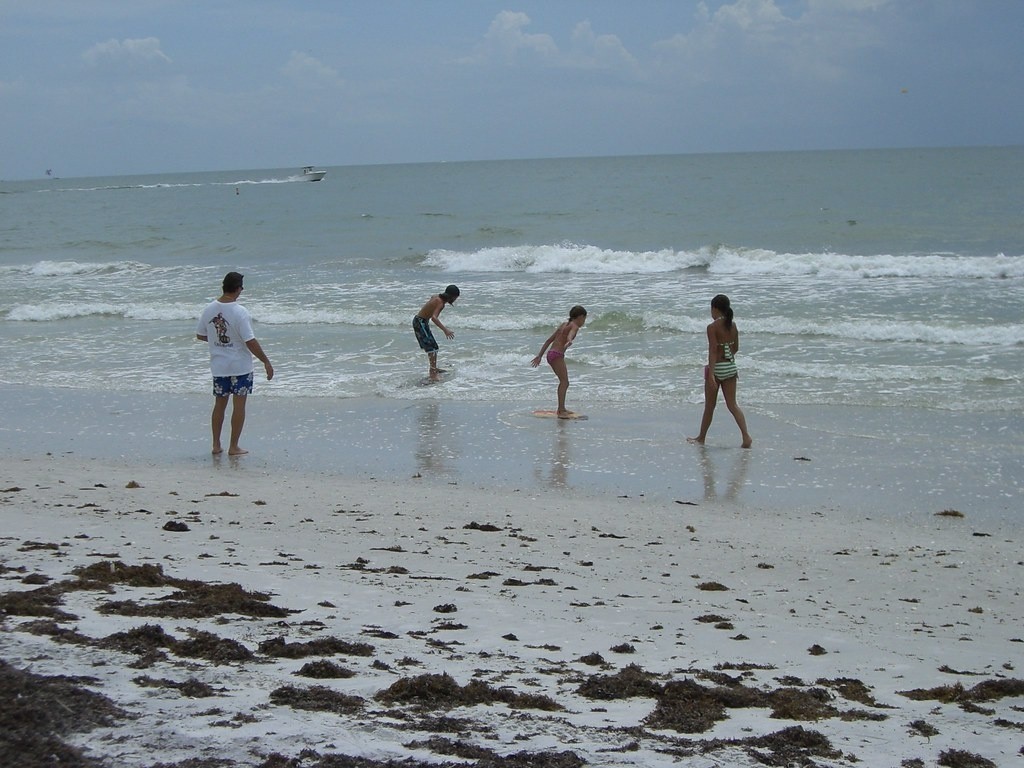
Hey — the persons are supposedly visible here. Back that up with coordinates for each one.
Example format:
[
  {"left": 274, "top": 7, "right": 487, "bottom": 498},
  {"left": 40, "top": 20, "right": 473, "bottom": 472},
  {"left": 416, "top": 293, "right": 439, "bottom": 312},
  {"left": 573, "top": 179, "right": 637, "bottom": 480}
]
[
  {"left": 687, "top": 294, "right": 752, "bottom": 448},
  {"left": 413, "top": 285, "right": 460, "bottom": 382},
  {"left": 196, "top": 271, "right": 274, "bottom": 455},
  {"left": 530, "top": 305, "right": 587, "bottom": 419}
]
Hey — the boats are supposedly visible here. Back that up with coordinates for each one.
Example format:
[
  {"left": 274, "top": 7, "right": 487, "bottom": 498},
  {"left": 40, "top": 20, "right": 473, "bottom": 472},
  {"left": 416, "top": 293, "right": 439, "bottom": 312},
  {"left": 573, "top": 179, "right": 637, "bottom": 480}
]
[{"left": 295, "top": 165, "right": 327, "bottom": 181}]
